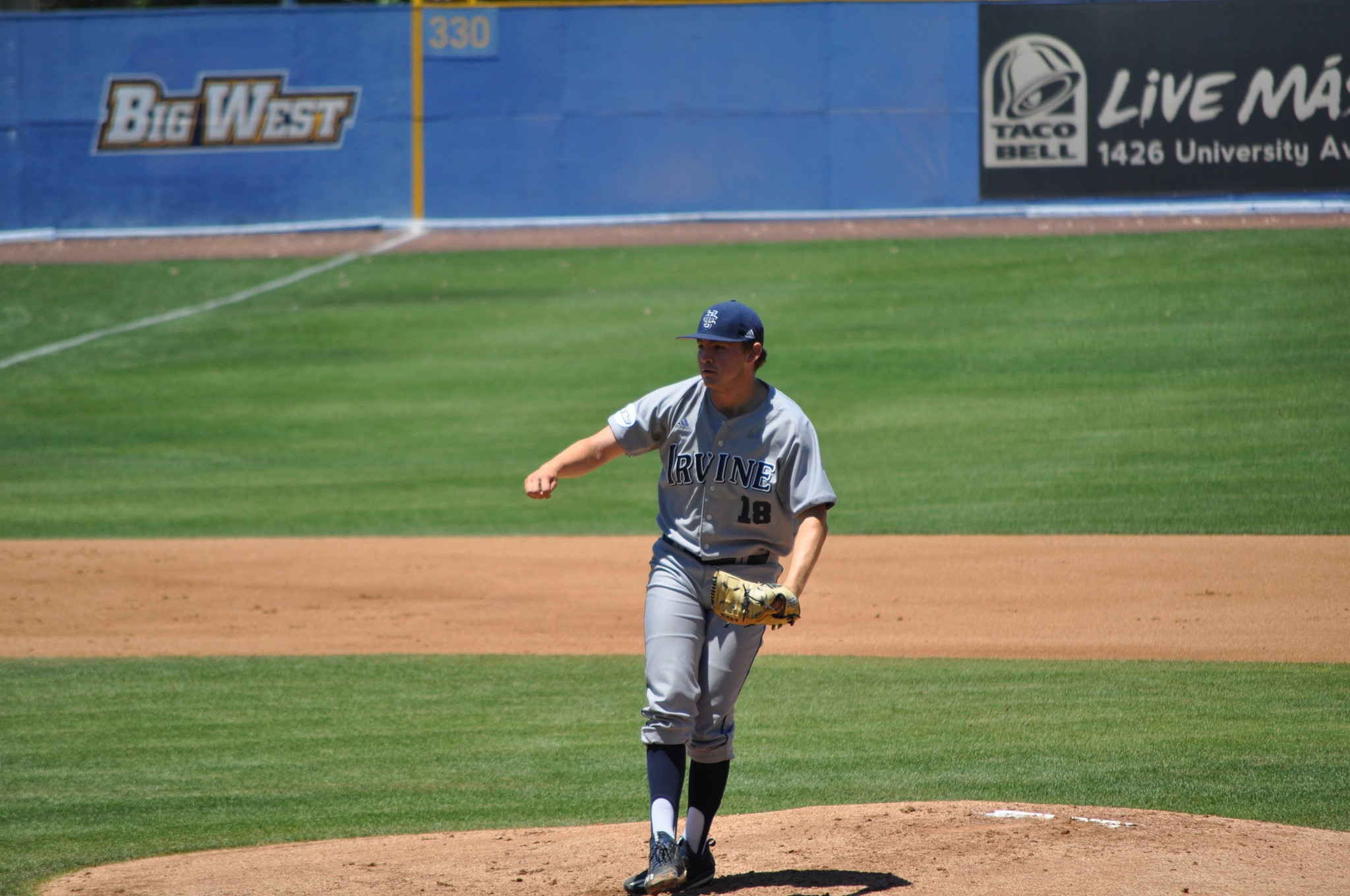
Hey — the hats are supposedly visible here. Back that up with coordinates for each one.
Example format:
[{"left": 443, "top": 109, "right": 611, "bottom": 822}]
[{"left": 675, "top": 300, "right": 764, "bottom": 346}]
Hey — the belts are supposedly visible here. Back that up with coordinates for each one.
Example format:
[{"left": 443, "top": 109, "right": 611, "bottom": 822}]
[{"left": 663, "top": 534, "right": 770, "bottom": 565}]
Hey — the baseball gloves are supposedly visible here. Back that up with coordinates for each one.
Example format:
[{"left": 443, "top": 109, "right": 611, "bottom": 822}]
[{"left": 710, "top": 567, "right": 800, "bottom": 627}]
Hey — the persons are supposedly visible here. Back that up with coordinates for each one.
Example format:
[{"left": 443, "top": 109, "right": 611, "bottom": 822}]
[{"left": 523, "top": 299, "right": 837, "bottom": 896}]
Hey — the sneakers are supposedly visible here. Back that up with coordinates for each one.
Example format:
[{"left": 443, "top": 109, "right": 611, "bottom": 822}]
[
  {"left": 644, "top": 831, "right": 687, "bottom": 891},
  {"left": 624, "top": 835, "right": 716, "bottom": 895}
]
